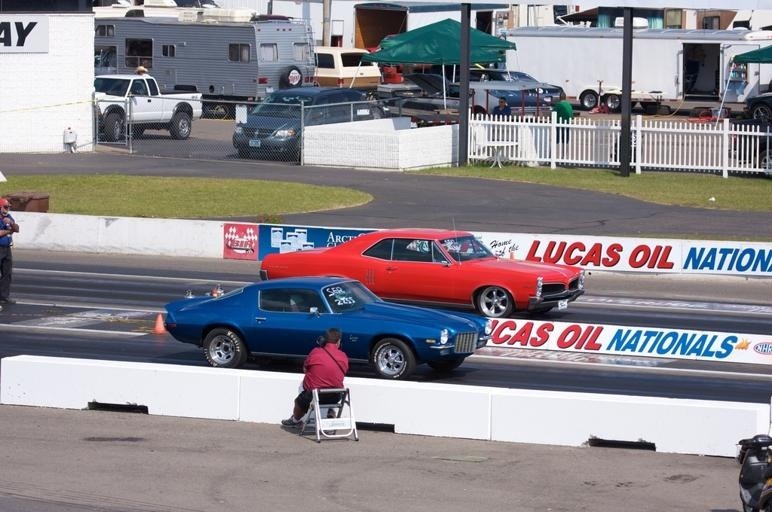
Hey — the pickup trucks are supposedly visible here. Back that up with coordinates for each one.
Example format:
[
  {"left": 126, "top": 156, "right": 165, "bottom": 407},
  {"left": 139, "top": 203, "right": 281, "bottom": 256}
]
[{"left": 92, "top": 66, "right": 207, "bottom": 144}]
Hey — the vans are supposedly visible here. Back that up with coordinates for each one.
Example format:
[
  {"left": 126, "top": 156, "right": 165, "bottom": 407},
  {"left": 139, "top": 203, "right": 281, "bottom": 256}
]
[{"left": 311, "top": 41, "right": 382, "bottom": 94}]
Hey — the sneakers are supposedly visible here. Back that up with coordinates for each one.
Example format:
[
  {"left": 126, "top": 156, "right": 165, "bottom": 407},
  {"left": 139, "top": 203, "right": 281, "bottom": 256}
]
[
  {"left": 327, "top": 409, "right": 336, "bottom": 418},
  {"left": 281, "top": 416, "right": 303, "bottom": 428}
]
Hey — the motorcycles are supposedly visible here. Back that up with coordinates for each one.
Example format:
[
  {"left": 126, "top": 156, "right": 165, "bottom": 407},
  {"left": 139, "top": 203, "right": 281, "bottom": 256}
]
[{"left": 734, "top": 431, "right": 771, "bottom": 512}]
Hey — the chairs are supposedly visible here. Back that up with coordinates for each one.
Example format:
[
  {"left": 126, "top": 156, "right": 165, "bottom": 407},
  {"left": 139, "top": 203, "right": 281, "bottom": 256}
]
[{"left": 299, "top": 387, "right": 359, "bottom": 442}]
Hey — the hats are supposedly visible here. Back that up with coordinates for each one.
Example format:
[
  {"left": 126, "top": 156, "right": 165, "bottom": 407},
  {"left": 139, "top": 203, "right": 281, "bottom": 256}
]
[{"left": 0, "top": 199, "right": 12, "bottom": 207}]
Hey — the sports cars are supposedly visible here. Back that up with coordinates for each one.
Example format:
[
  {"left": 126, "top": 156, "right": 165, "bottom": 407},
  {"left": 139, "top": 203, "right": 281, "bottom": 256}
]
[
  {"left": 159, "top": 274, "right": 493, "bottom": 381},
  {"left": 255, "top": 225, "right": 598, "bottom": 320}
]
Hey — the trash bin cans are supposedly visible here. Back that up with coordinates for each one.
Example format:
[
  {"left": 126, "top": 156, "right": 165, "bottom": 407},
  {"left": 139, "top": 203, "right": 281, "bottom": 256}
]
[{"left": 6, "top": 191, "right": 49, "bottom": 213}]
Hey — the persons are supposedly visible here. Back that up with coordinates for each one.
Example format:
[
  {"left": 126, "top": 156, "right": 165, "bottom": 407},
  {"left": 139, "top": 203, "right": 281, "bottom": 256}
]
[
  {"left": 551, "top": 94, "right": 573, "bottom": 158},
  {"left": 281, "top": 329, "right": 349, "bottom": 427},
  {"left": 489, "top": 97, "right": 513, "bottom": 117},
  {"left": 1, "top": 198, "right": 17, "bottom": 311}
]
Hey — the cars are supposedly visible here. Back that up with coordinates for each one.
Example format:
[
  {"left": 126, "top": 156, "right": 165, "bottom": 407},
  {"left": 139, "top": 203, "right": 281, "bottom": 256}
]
[
  {"left": 727, "top": 117, "right": 772, "bottom": 178},
  {"left": 742, "top": 90, "right": 772, "bottom": 125}
]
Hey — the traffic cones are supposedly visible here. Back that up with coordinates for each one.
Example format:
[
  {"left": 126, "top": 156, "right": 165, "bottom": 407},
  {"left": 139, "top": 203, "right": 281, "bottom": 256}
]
[{"left": 150, "top": 311, "right": 166, "bottom": 334}]
